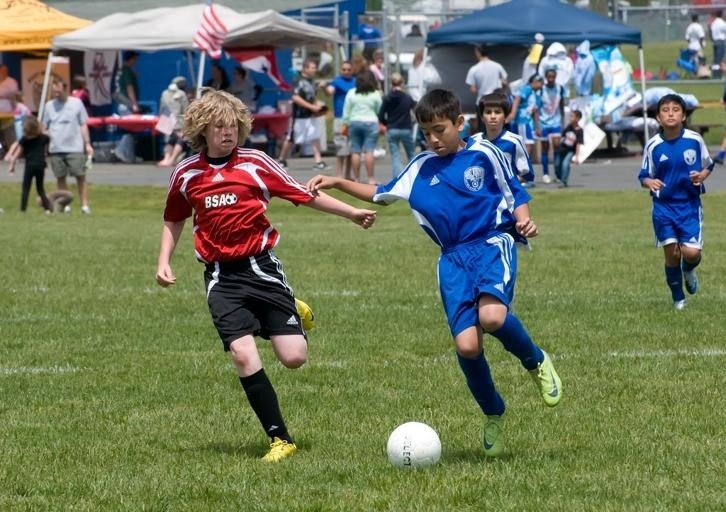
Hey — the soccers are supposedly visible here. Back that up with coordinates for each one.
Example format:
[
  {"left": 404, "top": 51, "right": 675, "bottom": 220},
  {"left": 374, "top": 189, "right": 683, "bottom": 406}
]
[{"left": 387, "top": 422, "right": 442, "bottom": 466}]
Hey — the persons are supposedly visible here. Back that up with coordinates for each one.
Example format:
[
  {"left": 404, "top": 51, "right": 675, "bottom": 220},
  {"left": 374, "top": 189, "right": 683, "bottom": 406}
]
[
  {"left": 155, "top": 90, "right": 377, "bottom": 461},
  {"left": 684, "top": 12, "right": 706, "bottom": 68},
  {"left": 306, "top": 89, "right": 563, "bottom": 456},
  {"left": 226, "top": 66, "right": 258, "bottom": 109},
  {"left": 713, "top": 89, "right": 726, "bottom": 166},
  {"left": 354, "top": 16, "right": 384, "bottom": 57},
  {"left": 637, "top": 93, "right": 716, "bottom": 310},
  {"left": 108, "top": 51, "right": 147, "bottom": 164},
  {"left": 709, "top": 9, "right": 726, "bottom": 65},
  {"left": 5, "top": 111, "right": 51, "bottom": 214},
  {"left": 10, "top": 93, "right": 32, "bottom": 140},
  {"left": 274, "top": 42, "right": 584, "bottom": 189},
  {"left": 405, "top": 25, "right": 423, "bottom": 38},
  {"left": 0, "top": 63, "right": 18, "bottom": 114},
  {"left": 156, "top": 78, "right": 185, "bottom": 166},
  {"left": 40, "top": 80, "right": 94, "bottom": 214},
  {"left": 204, "top": 63, "right": 230, "bottom": 90}
]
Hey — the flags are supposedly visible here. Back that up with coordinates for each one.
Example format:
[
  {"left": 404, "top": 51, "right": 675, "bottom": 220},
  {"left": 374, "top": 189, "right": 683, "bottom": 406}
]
[
  {"left": 193, "top": 2, "right": 226, "bottom": 58},
  {"left": 234, "top": 45, "right": 290, "bottom": 91}
]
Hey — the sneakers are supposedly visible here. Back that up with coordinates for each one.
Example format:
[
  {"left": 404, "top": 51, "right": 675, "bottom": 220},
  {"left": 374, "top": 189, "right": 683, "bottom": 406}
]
[
  {"left": 543, "top": 174, "right": 550, "bottom": 183},
  {"left": 684, "top": 268, "right": 697, "bottom": 294},
  {"left": 294, "top": 298, "right": 315, "bottom": 331},
  {"left": 554, "top": 178, "right": 561, "bottom": 183},
  {"left": 262, "top": 436, "right": 296, "bottom": 463},
  {"left": 482, "top": 410, "right": 505, "bottom": 456},
  {"left": 65, "top": 206, "right": 71, "bottom": 212},
  {"left": 279, "top": 160, "right": 287, "bottom": 167},
  {"left": 674, "top": 300, "right": 685, "bottom": 311},
  {"left": 521, "top": 182, "right": 534, "bottom": 189},
  {"left": 559, "top": 183, "right": 566, "bottom": 189},
  {"left": 82, "top": 205, "right": 90, "bottom": 213},
  {"left": 314, "top": 163, "right": 330, "bottom": 172},
  {"left": 529, "top": 348, "right": 562, "bottom": 407},
  {"left": 45, "top": 211, "right": 52, "bottom": 214}
]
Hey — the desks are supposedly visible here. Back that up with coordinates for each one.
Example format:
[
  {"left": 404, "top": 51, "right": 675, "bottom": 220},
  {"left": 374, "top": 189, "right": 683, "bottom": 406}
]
[{"left": 87, "top": 110, "right": 292, "bottom": 164}]
